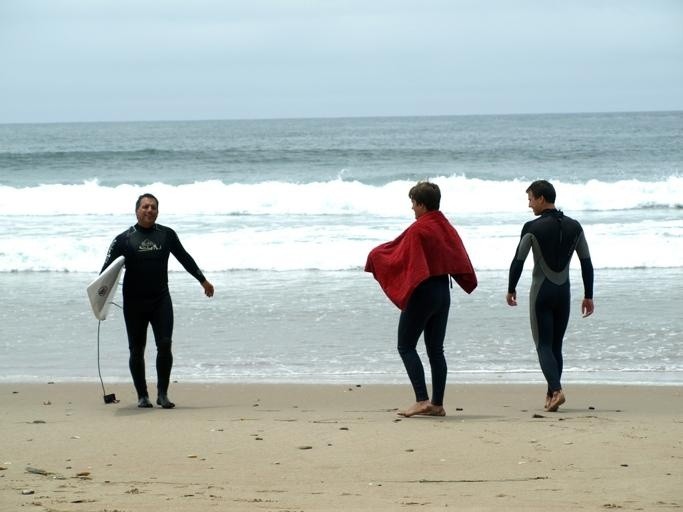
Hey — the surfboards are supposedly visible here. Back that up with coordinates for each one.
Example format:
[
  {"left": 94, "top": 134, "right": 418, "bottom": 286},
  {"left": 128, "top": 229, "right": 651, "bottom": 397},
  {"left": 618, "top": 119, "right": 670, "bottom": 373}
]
[{"left": 87, "top": 256, "right": 125, "bottom": 320}]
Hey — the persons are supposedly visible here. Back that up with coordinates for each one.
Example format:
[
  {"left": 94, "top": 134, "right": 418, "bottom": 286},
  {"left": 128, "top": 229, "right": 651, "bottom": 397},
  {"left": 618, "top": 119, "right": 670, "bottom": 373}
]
[
  {"left": 363, "top": 179, "right": 477, "bottom": 418},
  {"left": 505, "top": 179, "right": 594, "bottom": 414},
  {"left": 98, "top": 193, "right": 215, "bottom": 410}
]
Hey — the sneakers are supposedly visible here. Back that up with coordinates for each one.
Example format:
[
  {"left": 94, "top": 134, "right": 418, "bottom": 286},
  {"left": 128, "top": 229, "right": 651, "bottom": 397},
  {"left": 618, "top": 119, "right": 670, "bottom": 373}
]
[
  {"left": 137, "top": 392, "right": 153, "bottom": 407},
  {"left": 156, "top": 393, "right": 175, "bottom": 408}
]
